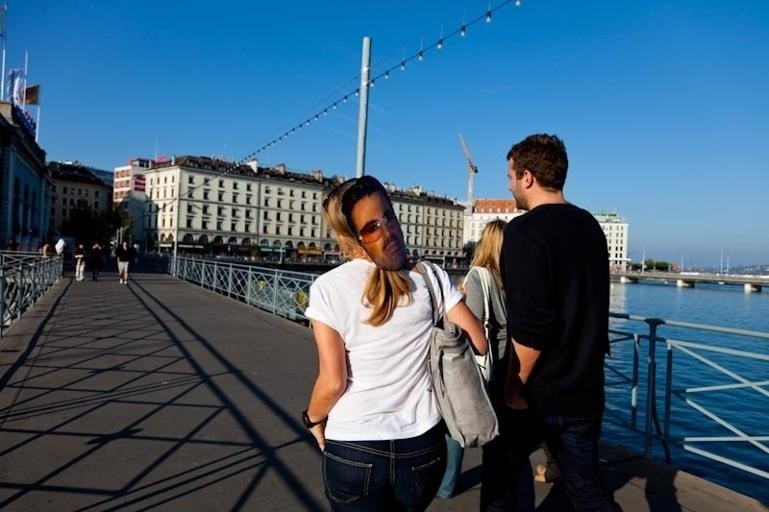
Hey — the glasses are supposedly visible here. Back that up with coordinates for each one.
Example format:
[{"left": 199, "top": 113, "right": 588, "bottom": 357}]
[{"left": 359, "top": 213, "right": 397, "bottom": 244}]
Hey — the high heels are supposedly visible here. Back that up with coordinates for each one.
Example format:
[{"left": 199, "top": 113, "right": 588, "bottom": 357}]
[{"left": 534, "top": 463, "right": 557, "bottom": 482}]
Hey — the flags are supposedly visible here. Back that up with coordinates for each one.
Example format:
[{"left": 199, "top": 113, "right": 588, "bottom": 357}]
[
  {"left": 5, "top": 68, "right": 24, "bottom": 108},
  {"left": 25, "top": 84, "right": 38, "bottom": 105}
]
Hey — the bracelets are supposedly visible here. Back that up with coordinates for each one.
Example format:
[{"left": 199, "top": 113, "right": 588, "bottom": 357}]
[{"left": 302, "top": 408, "right": 328, "bottom": 429}]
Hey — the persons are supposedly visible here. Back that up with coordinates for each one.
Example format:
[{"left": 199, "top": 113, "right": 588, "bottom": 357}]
[
  {"left": 435, "top": 220, "right": 510, "bottom": 500},
  {"left": 533, "top": 443, "right": 560, "bottom": 483},
  {"left": 39, "top": 237, "right": 141, "bottom": 286},
  {"left": 480, "top": 132, "right": 623, "bottom": 511},
  {"left": 343, "top": 176, "right": 407, "bottom": 271},
  {"left": 303, "top": 176, "right": 488, "bottom": 512},
  {"left": 181, "top": 250, "right": 339, "bottom": 265}
]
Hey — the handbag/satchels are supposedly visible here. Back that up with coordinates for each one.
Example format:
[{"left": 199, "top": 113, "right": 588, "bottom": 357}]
[{"left": 427, "top": 319, "right": 500, "bottom": 448}]
